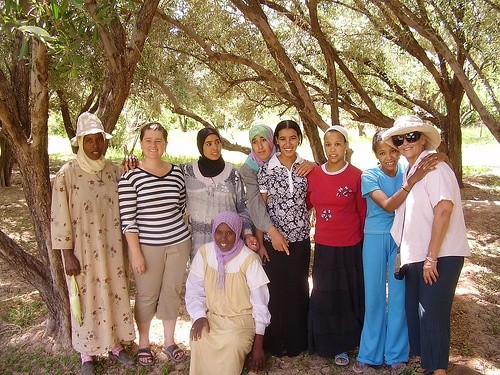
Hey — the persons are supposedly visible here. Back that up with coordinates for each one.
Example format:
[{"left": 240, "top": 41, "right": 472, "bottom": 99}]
[
  {"left": 185, "top": 211, "right": 272, "bottom": 375},
  {"left": 240, "top": 125, "right": 320, "bottom": 256},
  {"left": 383, "top": 115, "right": 472, "bottom": 375},
  {"left": 256, "top": 120, "right": 312, "bottom": 359},
  {"left": 50, "top": 111, "right": 138, "bottom": 375},
  {"left": 118, "top": 123, "right": 193, "bottom": 366},
  {"left": 353, "top": 131, "right": 453, "bottom": 375},
  {"left": 306, "top": 125, "right": 367, "bottom": 365},
  {"left": 123, "top": 127, "right": 260, "bottom": 265}
]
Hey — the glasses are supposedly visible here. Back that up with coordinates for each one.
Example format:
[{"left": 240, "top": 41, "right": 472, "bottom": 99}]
[{"left": 392, "top": 130, "right": 422, "bottom": 146}]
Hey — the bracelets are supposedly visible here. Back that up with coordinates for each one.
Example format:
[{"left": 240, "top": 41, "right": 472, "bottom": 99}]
[
  {"left": 425, "top": 255, "right": 438, "bottom": 263},
  {"left": 401, "top": 185, "right": 409, "bottom": 193}
]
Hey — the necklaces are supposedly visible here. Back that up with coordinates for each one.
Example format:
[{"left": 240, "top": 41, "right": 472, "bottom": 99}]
[{"left": 95, "top": 170, "right": 105, "bottom": 185}]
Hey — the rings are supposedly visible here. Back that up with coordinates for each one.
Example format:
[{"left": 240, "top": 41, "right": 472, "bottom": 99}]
[{"left": 191, "top": 328, "right": 195, "bottom": 330}]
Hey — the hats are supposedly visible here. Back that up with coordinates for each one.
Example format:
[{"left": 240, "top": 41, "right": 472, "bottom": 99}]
[
  {"left": 70, "top": 110, "right": 113, "bottom": 147},
  {"left": 382, "top": 115, "right": 441, "bottom": 152}
]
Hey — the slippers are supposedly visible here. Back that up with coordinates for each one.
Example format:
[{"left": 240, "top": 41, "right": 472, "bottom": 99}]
[
  {"left": 335, "top": 352, "right": 349, "bottom": 366},
  {"left": 162, "top": 344, "right": 186, "bottom": 362},
  {"left": 137, "top": 347, "right": 155, "bottom": 366}
]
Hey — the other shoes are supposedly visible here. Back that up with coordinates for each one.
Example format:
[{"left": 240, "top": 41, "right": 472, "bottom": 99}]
[
  {"left": 353, "top": 361, "right": 368, "bottom": 373},
  {"left": 81, "top": 359, "right": 95, "bottom": 375},
  {"left": 111, "top": 349, "right": 135, "bottom": 365},
  {"left": 391, "top": 362, "right": 406, "bottom": 375}
]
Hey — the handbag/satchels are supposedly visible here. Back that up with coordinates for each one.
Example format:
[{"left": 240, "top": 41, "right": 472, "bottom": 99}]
[{"left": 394, "top": 253, "right": 405, "bottom": 280}]
